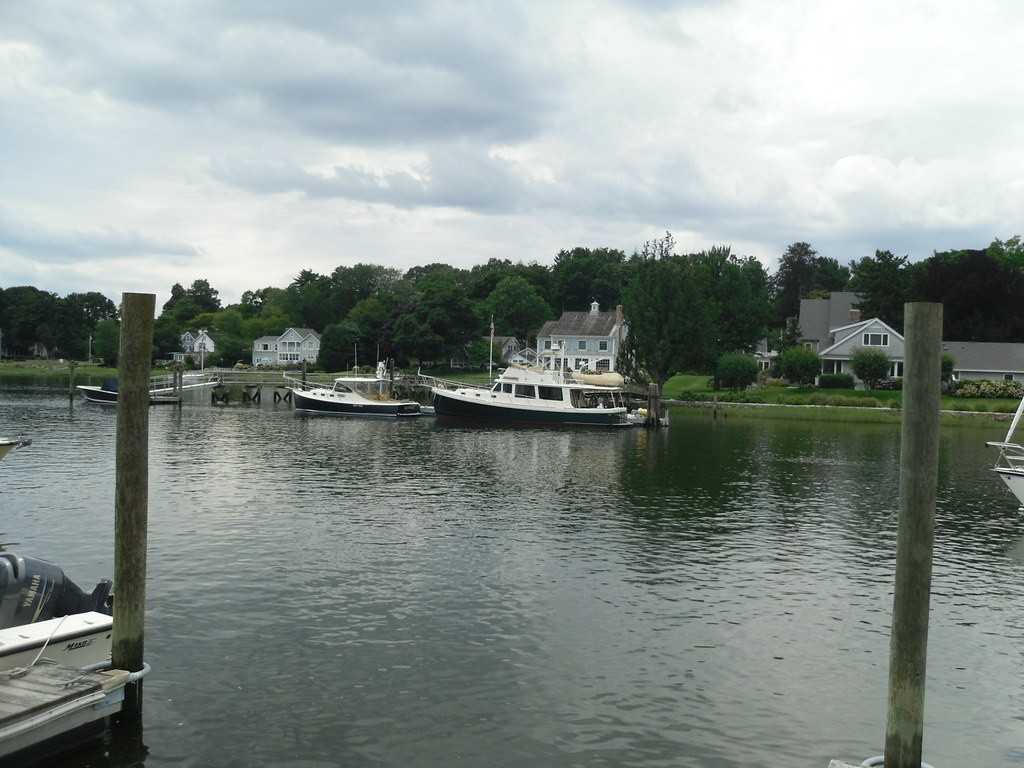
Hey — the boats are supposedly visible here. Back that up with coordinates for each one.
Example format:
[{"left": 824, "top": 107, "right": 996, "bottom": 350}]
[
  {"left": 283, "top": 343, "right": 422, "bottom": 419},
  {"left": 431, "top": 316, "right": 672, "bottom": 429},
  {"left": 75, "top": 327, "right": 220, "bottom": 406},
  {"left": 985, "top": 395, "right": 1024, "bottom": 512}
]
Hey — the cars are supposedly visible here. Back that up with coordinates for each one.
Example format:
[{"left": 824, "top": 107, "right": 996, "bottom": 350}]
[{"left": 234, "top": 360, "right": 254, "bottom": 370}]
